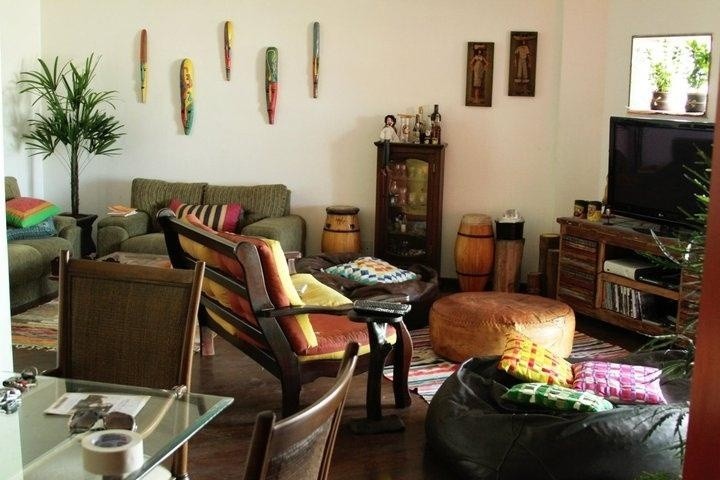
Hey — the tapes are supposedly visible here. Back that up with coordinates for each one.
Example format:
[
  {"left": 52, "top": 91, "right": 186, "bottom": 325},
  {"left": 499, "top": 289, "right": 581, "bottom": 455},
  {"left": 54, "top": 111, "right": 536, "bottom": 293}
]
[{"left": 80, "top": 428, "right": 144, "bottom": 476}]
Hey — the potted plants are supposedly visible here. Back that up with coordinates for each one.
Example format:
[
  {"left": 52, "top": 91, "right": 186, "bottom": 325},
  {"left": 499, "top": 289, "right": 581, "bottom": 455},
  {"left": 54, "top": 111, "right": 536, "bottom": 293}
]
[
  {"left": 17, "top": 49, "right": 129, "bottom": 276},
  {"left": 684, "top": 41, "right": 712, "bottom": 112},
  {"left": 645, "top": 40, "right": 681, "bottom": 111}
]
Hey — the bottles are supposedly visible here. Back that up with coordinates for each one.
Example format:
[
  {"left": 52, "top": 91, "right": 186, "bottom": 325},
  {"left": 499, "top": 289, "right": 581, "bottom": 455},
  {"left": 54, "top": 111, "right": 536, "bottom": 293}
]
[
  {"left": 432, "top": 114, "right": 442, "bottom": 143},
  {"left": 413, "top": 114, "right": 421, "bottom": 143},
  {"left": 419, "top": 106, "right": 428, "bottom": 140},
  {"left": 430, "top": 104, "right": 441, "bottom": 142}
]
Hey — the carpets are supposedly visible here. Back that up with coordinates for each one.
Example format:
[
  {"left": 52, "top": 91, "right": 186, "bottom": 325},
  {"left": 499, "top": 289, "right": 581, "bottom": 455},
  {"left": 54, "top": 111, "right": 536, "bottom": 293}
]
[
  {"left": 11, "top": 294, "right": 220, "bottom": 355},
  {"left": 358, "top": 326, "right": 632, "bottom": 407}
]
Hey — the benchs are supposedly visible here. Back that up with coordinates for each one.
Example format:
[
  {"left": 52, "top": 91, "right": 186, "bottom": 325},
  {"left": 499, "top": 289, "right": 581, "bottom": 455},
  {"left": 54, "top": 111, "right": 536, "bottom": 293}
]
[{"left": 154, "top": 214, "right": 415, "bottom": 420}]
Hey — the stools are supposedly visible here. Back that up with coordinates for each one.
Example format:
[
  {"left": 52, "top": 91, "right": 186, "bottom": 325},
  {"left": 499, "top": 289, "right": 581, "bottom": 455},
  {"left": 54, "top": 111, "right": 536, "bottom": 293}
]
[{"left": 428, "top": 290, "right": 576, "bottom": 363}]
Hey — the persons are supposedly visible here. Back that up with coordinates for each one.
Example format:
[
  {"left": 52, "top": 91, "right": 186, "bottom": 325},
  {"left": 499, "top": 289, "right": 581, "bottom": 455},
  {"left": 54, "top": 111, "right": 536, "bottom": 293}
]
[
  {"left": 513, "top": 41, "right": 532, "bottom": 84},
  {"left": 469, "top": 48, "right": 489, "bottom": 102},
  {"left": 379, "top": 114, "right": 399, "bottom": 142}
]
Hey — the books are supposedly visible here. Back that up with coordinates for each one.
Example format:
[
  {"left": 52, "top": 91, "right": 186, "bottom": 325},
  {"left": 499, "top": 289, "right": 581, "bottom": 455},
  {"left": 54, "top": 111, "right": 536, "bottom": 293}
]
[
  {"left": 107, "top": 206, "right": 138, "bottom": 217},
  {"left": 602, "top": 281, "right": 665, "bottom": 327}
]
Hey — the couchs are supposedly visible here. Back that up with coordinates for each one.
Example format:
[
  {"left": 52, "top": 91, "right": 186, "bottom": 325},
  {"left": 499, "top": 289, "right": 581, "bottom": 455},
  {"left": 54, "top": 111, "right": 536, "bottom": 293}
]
[
  {"left": 96, "top": 176, "right": 305, "bottom": 259},
  {"left": 4, "top": 176, "right": 77, "bottom": 309},
  {"left": 419, "top": 347, "right": 695, "bottom": 478},
  {"left": 297, "top": 250, "right": 441, "bottom": 331}
]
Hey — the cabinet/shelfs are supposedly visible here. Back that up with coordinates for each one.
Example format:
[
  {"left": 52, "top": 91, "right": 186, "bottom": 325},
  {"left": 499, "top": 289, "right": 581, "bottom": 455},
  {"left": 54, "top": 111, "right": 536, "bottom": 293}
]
[
  {"left": 373, "top": 138, "right": 448, "bottom": 309},
  {"left": 557, "top": 215, "right": 705, "bottom": 348}
]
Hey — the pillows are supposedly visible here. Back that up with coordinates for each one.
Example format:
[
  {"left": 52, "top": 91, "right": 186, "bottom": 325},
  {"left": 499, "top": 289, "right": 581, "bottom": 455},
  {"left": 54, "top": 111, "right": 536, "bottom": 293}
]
[
  {"left": 498, "top": 322, "right": 574, "bottom": 388},
  {"left": 570, "top": 360, "right": 669, "bottom": 406},
  {"left": 6, "top": 197, "right": 63, "bottom": 228},
  {"left": 499, "top": 382, "right": 615, "bottom": 412},
  {"left": 166, "top": 198, "right": 244, "bottom": 233},
  {"left": 6, "top": 215, "right": 59, "bottom": 239},
  {"left": 320, "top": 256, "right": 423, "bottom": 288}
]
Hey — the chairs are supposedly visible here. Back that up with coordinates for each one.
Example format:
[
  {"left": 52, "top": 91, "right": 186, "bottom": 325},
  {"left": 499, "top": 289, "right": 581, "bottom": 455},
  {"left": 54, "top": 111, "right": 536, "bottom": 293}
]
[
  {"left": 237, "top": 342, "right": 359, "bottom": 480},
  {"left": 37, "top": 249, "right": 206, "bottom": 480}
]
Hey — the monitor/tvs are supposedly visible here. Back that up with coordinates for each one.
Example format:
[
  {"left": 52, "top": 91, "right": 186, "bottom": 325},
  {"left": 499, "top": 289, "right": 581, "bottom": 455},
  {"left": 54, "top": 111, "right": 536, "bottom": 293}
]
[{"left": 605, "top": 116, "right": 716, "bottom": 238}]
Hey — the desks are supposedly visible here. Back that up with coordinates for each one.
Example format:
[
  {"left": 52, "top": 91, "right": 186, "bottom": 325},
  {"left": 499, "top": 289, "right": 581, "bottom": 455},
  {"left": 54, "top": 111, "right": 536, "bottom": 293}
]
[
  {"left": 0, "top": 370, "right": 235, "bottom": 480},
  {"left": 96, "top": 250, "right": 174, "bottom": 269}
]
